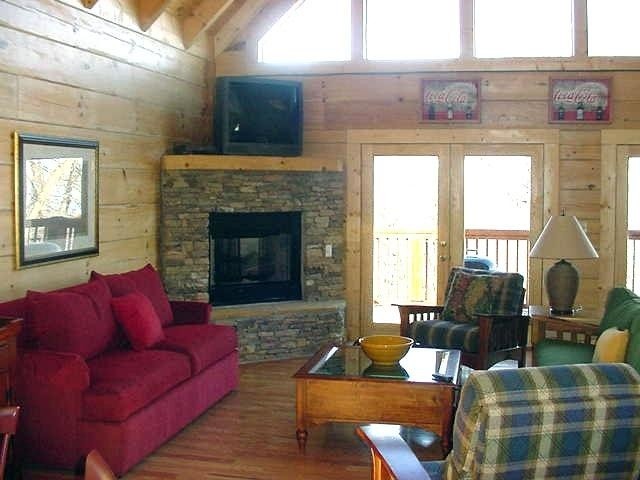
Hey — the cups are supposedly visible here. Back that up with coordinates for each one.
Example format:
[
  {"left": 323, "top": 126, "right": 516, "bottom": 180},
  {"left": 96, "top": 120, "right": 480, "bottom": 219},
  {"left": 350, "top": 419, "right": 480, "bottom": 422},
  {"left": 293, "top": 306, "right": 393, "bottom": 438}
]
[{"left": 528, "top": 303, "right": 547, "bottom": 319}]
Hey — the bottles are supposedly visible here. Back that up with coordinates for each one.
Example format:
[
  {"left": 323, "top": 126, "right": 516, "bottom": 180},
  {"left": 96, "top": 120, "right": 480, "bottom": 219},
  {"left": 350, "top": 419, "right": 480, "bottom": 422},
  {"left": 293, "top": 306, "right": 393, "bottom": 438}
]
[
  {"left": 427, "top": 101, "right": 473, "bottom": 120},
  {"left": 557, "top": 101, "right": 604, "bottom": 120}
]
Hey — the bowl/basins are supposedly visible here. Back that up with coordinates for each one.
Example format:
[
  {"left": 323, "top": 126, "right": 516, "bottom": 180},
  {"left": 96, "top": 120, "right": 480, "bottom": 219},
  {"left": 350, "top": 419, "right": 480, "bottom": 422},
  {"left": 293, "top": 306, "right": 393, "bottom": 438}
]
[
  {"left": 358, "top": 334, "right": 414, "bottom": 366},
  {"left": 356, "top": 366, "right": 410, "bottom": 380}
]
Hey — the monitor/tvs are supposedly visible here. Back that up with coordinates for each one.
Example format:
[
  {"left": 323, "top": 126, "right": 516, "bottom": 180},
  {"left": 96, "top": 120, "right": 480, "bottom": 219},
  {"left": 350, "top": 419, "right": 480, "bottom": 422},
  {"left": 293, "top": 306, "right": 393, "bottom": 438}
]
[{"left": 213, "top": 76, "right": 304, "bottom": 157}]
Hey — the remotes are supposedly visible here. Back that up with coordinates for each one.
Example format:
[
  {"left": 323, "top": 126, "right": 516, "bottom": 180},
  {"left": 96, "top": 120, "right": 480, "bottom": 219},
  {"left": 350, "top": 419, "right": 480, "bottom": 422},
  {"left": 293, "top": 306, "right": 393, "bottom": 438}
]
[{"left": 432, "top": 374, "right": 453, "bottom": 383}]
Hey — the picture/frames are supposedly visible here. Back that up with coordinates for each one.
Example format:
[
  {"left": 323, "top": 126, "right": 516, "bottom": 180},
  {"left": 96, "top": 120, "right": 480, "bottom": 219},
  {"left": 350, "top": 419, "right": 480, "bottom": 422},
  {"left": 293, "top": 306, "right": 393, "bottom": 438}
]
[
  {"left": 548, "top": 76, "right": 612, "bottom": 124},
  {"left": 419, "top": 79, "right": 480, "bottom": 123},
  {"left": 14, "top": 130, "right": 99, "bottom": 270}
]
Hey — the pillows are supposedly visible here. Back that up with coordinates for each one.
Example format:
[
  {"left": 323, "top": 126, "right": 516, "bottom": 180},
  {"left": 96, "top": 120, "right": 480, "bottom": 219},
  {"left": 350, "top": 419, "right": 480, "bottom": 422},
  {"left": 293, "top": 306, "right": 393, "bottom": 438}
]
[
  {"left": 24, "top": 277, "right": 119, "bottom": 360},
  {"left": 441, "top": 270, "right": 502, "bottom": 326},
  {"left": 110, "top": 290, "right": 166, "bottom": 352},
  {"left": 592, "top": 326, "right": 629, "bottom": 362},
  {"left": 91, "top": 263, "right": 174, "bottom": 327}
]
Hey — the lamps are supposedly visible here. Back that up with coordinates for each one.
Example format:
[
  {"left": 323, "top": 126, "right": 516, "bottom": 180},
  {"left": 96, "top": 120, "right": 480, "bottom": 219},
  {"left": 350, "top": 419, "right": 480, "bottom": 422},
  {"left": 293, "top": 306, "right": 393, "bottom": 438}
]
[{"left": 528, "top": 208, "right": 600, "bottom": 315}]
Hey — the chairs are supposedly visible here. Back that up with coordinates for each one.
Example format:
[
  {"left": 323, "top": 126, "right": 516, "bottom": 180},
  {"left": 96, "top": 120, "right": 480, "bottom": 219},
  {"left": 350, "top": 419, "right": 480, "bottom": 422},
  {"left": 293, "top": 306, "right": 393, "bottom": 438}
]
[
  {"left": 0, "top": 406, "right": 19, "bottom": 480},
  {"left": 391, "top": 266, "right": 529, "bottom": 370},
  {"left": 355, "top": 363, "right": 640, "bottom": 479},
  {"left": 533, "top": 286, "right": 640, "bottom": 374}
]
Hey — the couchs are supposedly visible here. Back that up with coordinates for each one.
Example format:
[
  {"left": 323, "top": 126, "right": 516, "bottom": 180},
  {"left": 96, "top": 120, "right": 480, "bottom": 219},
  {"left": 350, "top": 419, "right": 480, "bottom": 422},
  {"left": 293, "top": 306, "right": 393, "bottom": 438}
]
[{"left": 0, "top": 283, "right": 239, "bottom": 475}]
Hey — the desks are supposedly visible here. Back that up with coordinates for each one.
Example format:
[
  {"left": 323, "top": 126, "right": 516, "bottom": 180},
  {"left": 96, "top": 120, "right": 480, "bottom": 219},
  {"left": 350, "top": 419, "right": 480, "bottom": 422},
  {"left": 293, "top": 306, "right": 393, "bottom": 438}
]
[
  {"left": 529, "top": 305, "right": 600, "bottom": 347},
  {"left": 0, "top": 316, "right": 24, "bottom": 464}
]
